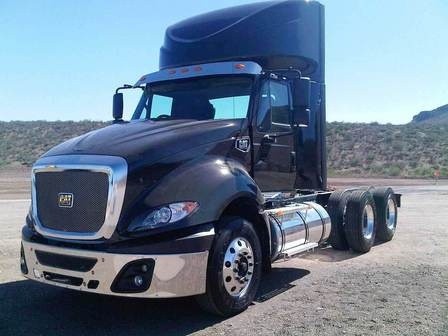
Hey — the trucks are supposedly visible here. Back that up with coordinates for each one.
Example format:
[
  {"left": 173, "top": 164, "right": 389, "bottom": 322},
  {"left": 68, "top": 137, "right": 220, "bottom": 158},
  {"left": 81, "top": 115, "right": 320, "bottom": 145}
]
[{"left": 18, "top": 0, "right": 402, "bottom": 319}]
